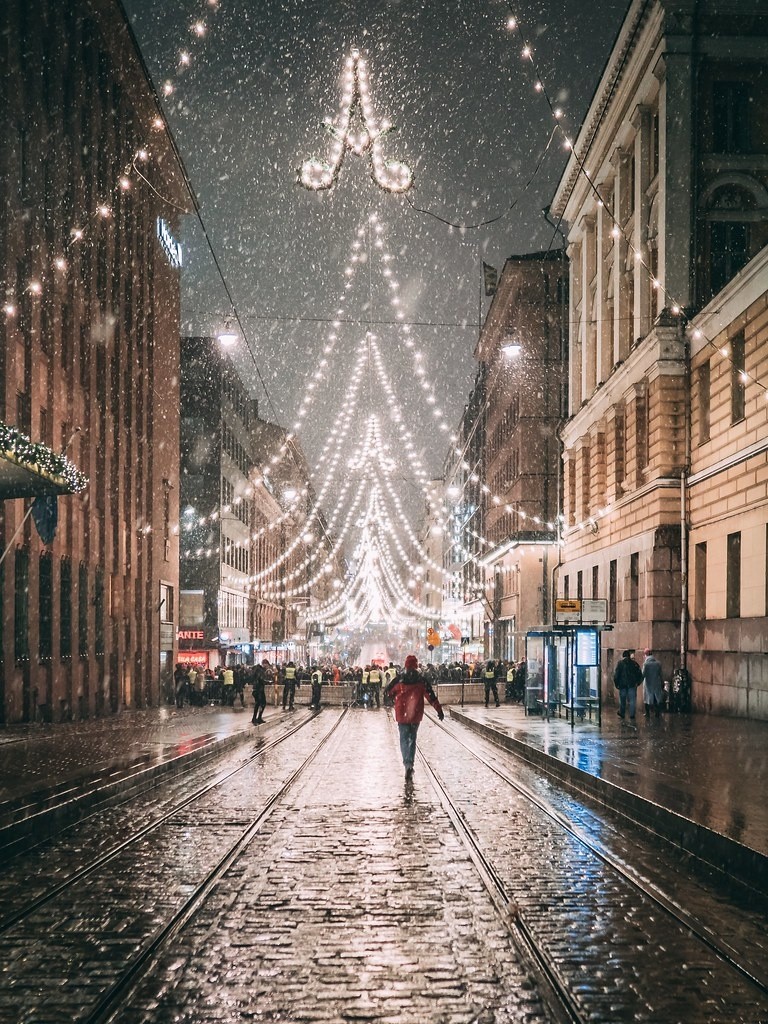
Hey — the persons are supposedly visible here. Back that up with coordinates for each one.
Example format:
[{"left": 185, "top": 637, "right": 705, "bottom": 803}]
[
  {"left": 640, "top": 648, "right": 665, "bottom": 720},
  {"left": 613, "top": 650, "right": 645, "bottom": 718},
  {"left": 174, "top": 658, "right": 531, "bottom": 711},
  {"left": 248, "top": 659, "right": 271, "bottom": 724},
  {"left": 385, "top": 655, "right": 444, "bottom": 781}
]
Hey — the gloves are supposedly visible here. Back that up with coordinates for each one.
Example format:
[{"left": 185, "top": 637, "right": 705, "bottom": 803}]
[
  {"left": 270, "top": 681, "right": 274, "bottom": 684},
  {"left": 438, "top": 712, "right": 444, "bottom": 721}
]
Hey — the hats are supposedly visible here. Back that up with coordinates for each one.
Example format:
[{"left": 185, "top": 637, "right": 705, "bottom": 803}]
[
  {"left": 623, "top": 651, "right": 630, "bottom": 658},
  {"left": 644, "top": 648, "right": 652, "bottom": 654},
  {"left": 263, "top": 659, "right": 269, "bottom": 665},
  {"left": 405, "top": 655, "right": 417, "bottom": 669}
]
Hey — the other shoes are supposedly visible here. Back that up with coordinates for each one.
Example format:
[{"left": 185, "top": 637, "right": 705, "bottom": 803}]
[
  {"left": 643, "top": 713, "right": 650, "bottom": 716},
  {"left": 617, "top": 712, "right": 625, "bottom": 720},
  {"left": 174, "top": 698, "right": 536, "bottom": 707},
  {"left": 654, "top": 713, "right": 660, "bottom": 717},
  {"left": 628, "top": 715, "right": 636, "bottom": 719},
  {"left": 252, "top": 719, "right": 267, "bottom": 723},
  {"left": 404, "top": 766, "right": 413, "bottom": 781}
]
